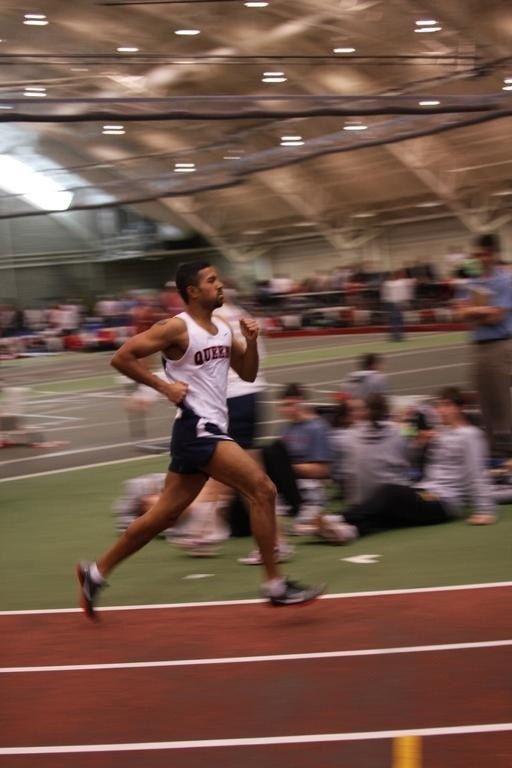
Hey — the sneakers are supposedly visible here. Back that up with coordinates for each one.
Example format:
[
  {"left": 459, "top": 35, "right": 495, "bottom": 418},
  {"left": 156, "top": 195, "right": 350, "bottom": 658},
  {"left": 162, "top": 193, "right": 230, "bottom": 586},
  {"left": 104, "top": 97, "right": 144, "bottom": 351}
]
[
  {"left": 75, "top": 561, "right": 109, "bottom": 623},
  {"left": 137, "top": 493, "right": 358, "bottom": 566},
  {"left": 270, "top": 578, "right": 326, "bottom": 605}
]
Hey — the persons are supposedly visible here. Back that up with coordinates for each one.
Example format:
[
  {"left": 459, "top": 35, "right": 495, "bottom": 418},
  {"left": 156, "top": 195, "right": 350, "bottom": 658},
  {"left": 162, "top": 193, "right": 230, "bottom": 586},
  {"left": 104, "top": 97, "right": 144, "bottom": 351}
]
[
  {"left": 452, "top": 231, "right": 511, "bottom": 451},
  {"left": 108, "top": 351, "right": 511, "bottom": 549},
  {"left": 118, "top": 299, "right": 299, "bottom": 566},
  {"left": 223, "top": 242, "right": 484, "bottom": 341},
  {"left": 72, "top": 259, "right": 329, "bottom": 620},
  {"left": 0, "top": 279, "right": 187, "bottom": 440}
]
